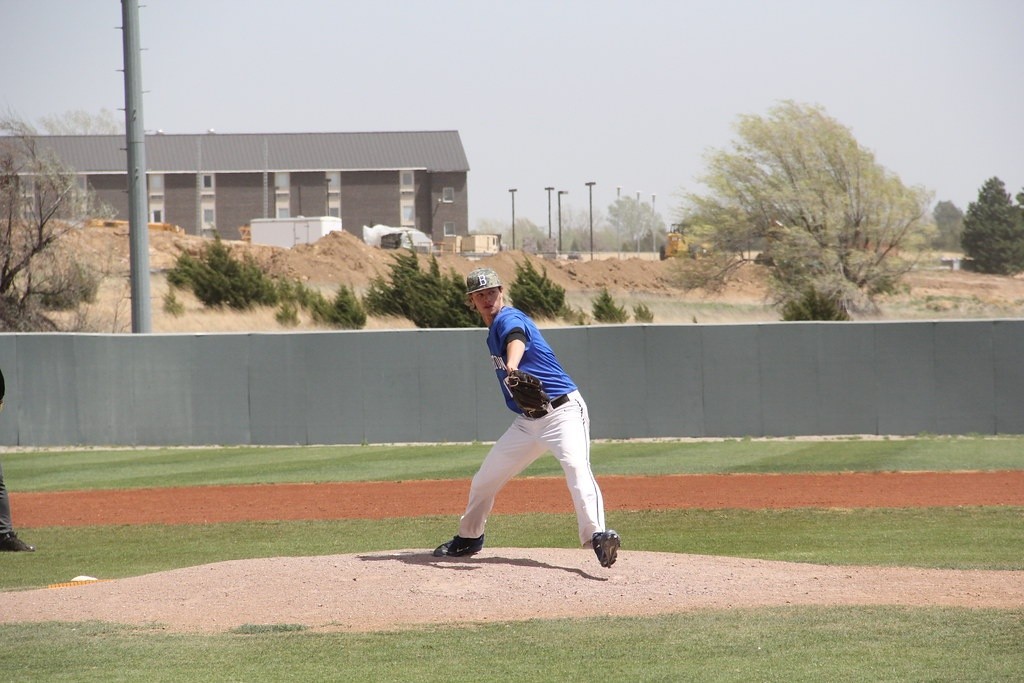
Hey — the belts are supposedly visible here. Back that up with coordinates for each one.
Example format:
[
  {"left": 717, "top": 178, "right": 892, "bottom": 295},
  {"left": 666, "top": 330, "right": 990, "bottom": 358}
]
[{"left": 521, "top": 394, "right": 569, "bottom": 420}]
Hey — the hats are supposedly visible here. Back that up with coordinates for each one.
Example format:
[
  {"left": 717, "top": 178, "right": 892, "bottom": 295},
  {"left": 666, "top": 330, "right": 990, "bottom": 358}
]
[{"left": 466, "top": 268, "right": 502, "bottom": 295}]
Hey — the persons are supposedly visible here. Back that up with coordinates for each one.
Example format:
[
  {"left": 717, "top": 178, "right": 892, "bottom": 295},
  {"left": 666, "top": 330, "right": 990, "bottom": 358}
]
[
  {"left": 431, "top": 267, "right": 621, "bottom": 568},
  {"left": 0, "top": 368, "right": 36, "bottom": 553}
]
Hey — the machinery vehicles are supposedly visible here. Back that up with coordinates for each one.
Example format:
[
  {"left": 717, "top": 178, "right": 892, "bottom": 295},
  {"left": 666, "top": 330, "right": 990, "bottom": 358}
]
[{"left": 660, "top": 223, "right": 701, "bottom": 261}]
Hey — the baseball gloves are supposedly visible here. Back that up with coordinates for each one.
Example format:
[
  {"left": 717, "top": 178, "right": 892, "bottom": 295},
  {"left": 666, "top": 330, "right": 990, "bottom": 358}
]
[{"left": 507, "top": 370, "right": 549, "bottom": 411}]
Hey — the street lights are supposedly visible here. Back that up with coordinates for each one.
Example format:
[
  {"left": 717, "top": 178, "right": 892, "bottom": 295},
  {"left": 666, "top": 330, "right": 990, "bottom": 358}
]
[
  {"left": 617, "top": 186, "right": 622, "bottom": 258},
  {"left": 557, "top": 190, "right": 568, "bottom": 252},
  {"left": 652, "top": 194, "right": 657, "bottom": 258},
  {"left": 509, "top": 189, "right": 517, "bottom": 248},
  {"left": 586, "top": 181, "right": 596, "bottom": 260},
  {"left": 636, "top": 191, "right": 642, "bottom": 258},
  {"left": 545, "top": 187, "right": 554, "bottom": 237}
]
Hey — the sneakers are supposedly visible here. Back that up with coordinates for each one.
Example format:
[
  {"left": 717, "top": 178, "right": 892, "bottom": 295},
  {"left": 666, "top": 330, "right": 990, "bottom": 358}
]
[
  {"left": 592, "top": 530, "right": 621, "bottom": 569},
  {"left": 432, "top": 534, "right": 484, "bottom": 557},
  {"left": 0, "top": 531, "right": 36, "bottom": 551}
]
